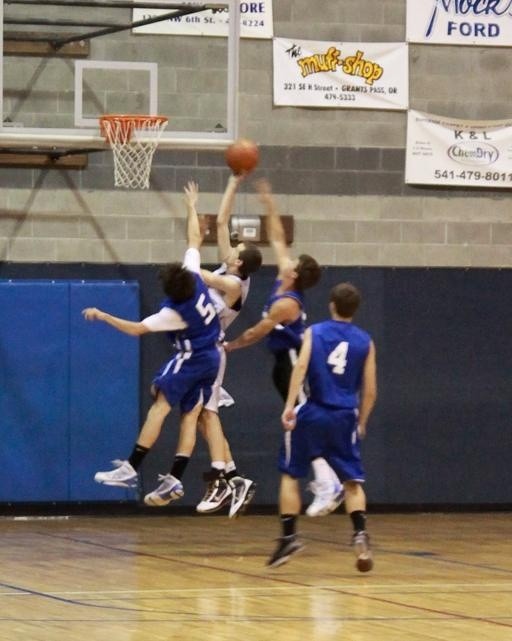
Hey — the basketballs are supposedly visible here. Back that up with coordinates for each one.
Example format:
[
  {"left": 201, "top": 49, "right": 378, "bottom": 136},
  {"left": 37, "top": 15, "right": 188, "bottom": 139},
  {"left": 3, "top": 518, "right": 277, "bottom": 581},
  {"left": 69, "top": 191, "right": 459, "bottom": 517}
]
[{"left": 225, "top": 141, "right": 258, "bottom": 173}]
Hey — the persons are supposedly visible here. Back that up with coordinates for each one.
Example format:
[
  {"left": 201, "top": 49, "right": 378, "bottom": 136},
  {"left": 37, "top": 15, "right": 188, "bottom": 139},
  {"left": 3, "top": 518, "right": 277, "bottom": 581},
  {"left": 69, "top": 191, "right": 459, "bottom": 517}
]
[
  {"left": 195, "top": 172, "right": 263, "bottom": 521},
  {"left": 266, "top": 282, "right": 378, "bottom": 573},
  {"left": 82, "top": 179, "right": 221, "bottom": 508},
  {"left": 221, "top": 182, "right": 346, "bottom": 517}
]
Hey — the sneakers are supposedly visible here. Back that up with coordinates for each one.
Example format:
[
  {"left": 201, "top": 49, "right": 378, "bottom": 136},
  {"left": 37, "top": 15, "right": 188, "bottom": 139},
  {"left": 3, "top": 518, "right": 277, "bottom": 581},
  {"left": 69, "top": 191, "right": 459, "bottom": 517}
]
[
  {"left": 144, "top": 474, "right": 184, "bottom": 507},
  {"left": 266, "top": 534, "right": 304, "bottom": 568},
  {"left": 196, "top": 468, "right": 257, "bottom": 518},
  {"left": 353, "top": 530, "right": 374, "bottom": 572},
  {"left": 94, "top": 459, "right": 138, "bottom": 487},
  {"left": 306, "top": 476, "right": 344, "bottom": 518}
]
